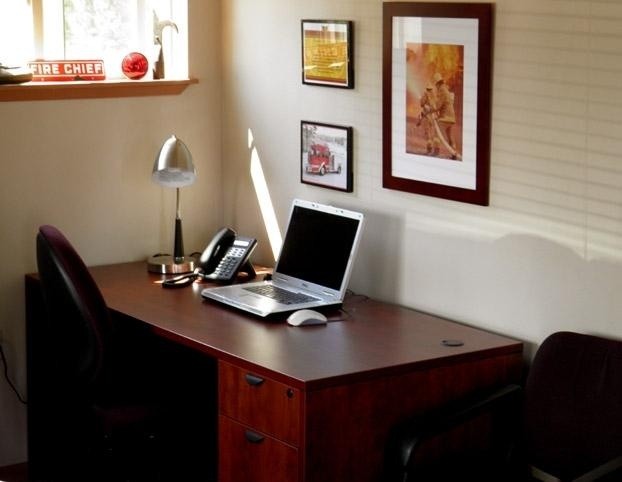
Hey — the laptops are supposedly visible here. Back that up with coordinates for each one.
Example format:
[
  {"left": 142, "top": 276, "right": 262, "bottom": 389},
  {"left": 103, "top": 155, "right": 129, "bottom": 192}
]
[{"left": 200, "top": 199, "right": 366, "bottom": 322}]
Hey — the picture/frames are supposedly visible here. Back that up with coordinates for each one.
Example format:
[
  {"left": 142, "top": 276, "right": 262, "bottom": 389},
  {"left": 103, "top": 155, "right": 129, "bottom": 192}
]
[
  {"left": 382, "top": 1, "right": 495, "bottom": 208},
  {"left": 301, "top": 19, "right": 354, "bottom": 90},
  {"left": 300, "top": 120, "right": 354, "bottom": 193}
]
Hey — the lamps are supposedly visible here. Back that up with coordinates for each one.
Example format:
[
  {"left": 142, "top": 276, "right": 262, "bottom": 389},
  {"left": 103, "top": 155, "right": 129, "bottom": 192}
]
[{"left": 146, "top": 135, "right": 196, "bottom": 273}]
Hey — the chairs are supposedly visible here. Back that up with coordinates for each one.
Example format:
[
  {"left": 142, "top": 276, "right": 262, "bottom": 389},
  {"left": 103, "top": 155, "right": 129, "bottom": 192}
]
[
  {"left": 384, "top": 331, "right": 621, "bottom": 481},
  {"left": 35, "top": 224, "right": 115, "bottom": 368}
]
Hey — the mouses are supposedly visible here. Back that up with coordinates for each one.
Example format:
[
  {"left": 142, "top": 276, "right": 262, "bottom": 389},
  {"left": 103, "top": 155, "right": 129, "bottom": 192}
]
[{"left": 287, "top": 308, "right": 328, "bottom": 327}]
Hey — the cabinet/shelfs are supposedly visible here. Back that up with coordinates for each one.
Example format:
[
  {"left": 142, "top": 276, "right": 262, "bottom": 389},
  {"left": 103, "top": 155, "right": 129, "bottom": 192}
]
[{"left": 24, "top": 258, "right": 526, "bottom": 482}]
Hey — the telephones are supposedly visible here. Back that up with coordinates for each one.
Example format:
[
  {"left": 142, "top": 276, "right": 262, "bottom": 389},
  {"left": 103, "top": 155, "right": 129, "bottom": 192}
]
[{"left": 161, "top": 227, "right": 258, "bottom": 290}]
